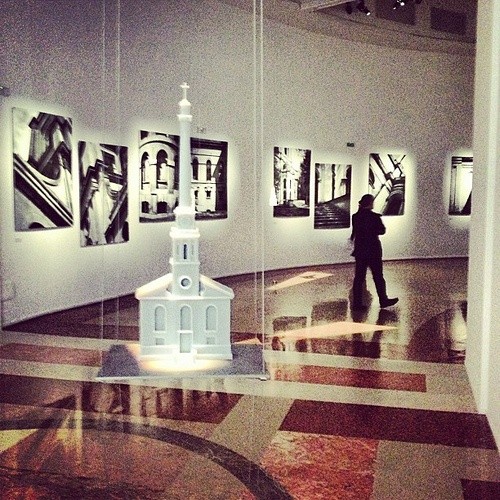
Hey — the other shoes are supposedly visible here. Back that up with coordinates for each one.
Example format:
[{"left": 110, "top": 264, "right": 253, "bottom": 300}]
[
  {"left": 380, "top": 297, "right": 399, "bottom": 309},
  {"left": 353, "top": 305, "right": 367, "bottom": 311}
]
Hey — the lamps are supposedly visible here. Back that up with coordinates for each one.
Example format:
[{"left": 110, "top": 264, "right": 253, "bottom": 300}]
[
  {"left": 393, "top": 0, "right": 406, "bottom": 10},
  {"left": 357, "top": 2, "right": 370, "bottom": 15}
]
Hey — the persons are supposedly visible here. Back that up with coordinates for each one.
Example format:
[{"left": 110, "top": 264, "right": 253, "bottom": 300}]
[{"left": 352, "top": 194, "right": 399, "bottom": 308}]
